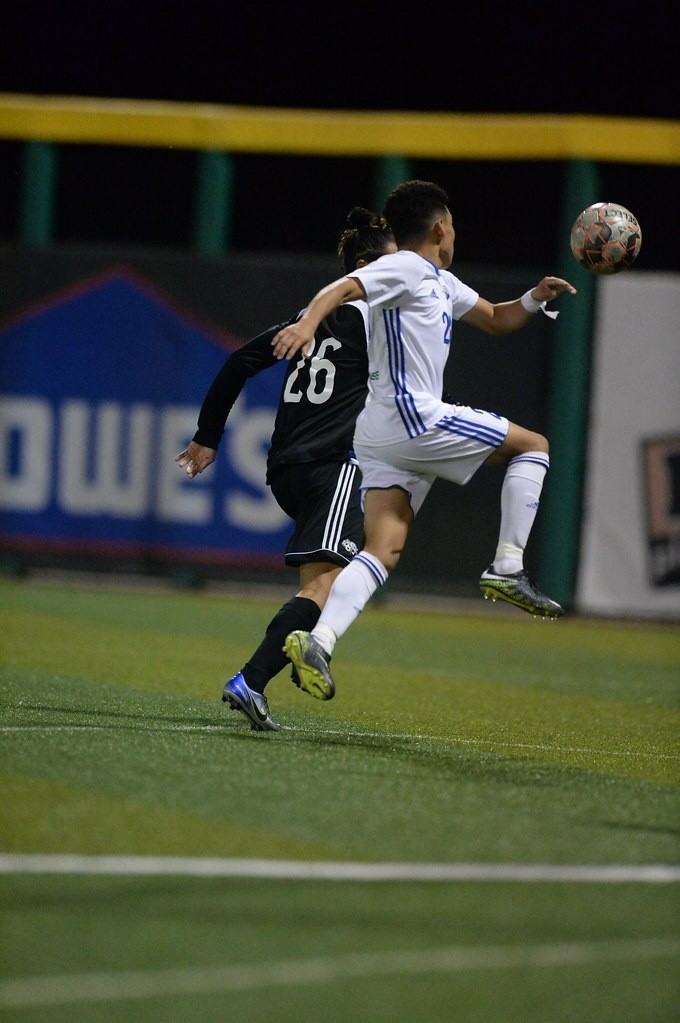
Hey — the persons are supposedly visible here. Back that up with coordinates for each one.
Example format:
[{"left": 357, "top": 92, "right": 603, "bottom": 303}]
[
  {"left": 177, "top": 212, "right": 402, "bottom": 730},
  {"left": 269, "top": 181, "right": 577, "bottom": 700}
]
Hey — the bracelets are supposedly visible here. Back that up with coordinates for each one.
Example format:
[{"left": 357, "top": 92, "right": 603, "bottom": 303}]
[{"left": 522, "top": 287, "right": 559, "bottom": 321}]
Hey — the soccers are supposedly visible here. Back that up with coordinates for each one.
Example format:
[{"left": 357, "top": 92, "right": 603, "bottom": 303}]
[{"left": 569, "top": 200, "right": 641, "bottom": 274}]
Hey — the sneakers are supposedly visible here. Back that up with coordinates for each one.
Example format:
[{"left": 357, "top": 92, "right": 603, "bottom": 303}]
[
  {"left": 479, "top": 565, "right": 564, "bottom": 619},
  {"left": 222, "top": 674, "right": 281, "bottom": 733},
  {"left": 282, "top": 631, "right": 335, "bottom": 701}
]
